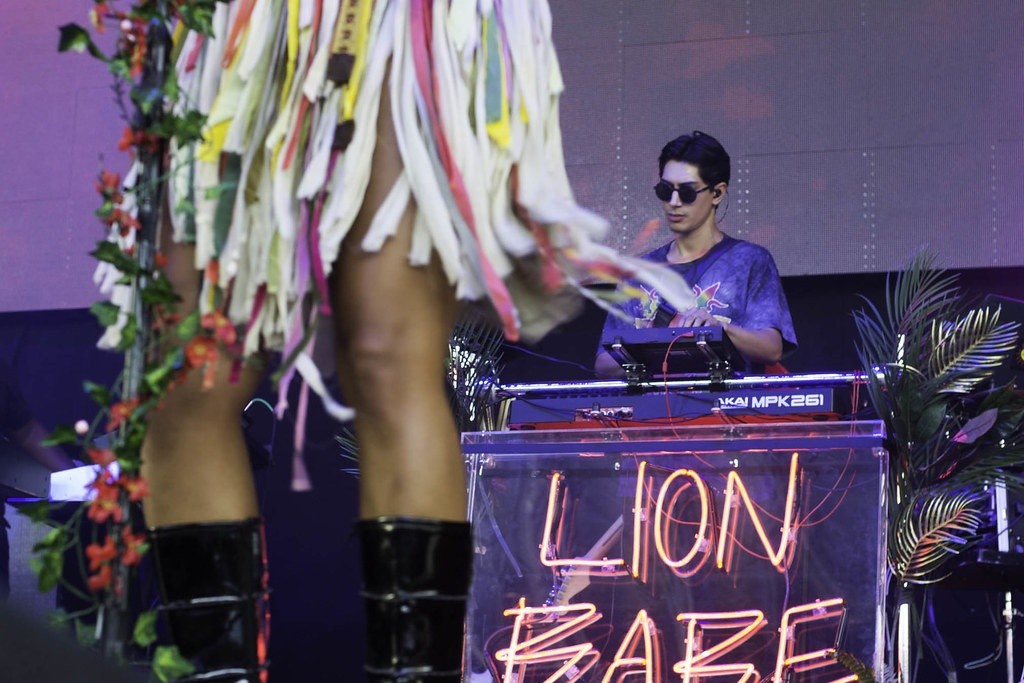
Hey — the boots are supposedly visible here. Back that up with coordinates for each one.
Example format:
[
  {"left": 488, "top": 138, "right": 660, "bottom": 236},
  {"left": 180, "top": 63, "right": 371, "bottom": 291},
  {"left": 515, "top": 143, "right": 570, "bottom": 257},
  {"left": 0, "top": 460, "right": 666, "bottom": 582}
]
[
  {"left": 146, "top": 513, "right": 259, "bottom": 683},
  {"left": 356, "top": 512, "right": 472, "bottom": 683}
]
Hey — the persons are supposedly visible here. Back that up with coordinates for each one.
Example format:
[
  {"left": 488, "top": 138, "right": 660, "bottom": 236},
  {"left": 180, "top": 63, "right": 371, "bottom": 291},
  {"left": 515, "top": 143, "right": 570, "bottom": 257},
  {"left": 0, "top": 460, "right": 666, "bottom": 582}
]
[
  {"left": 93, "top": 0, "right": 697, "bottom": 682},
  {"left": 593, "top": 129, "right": 799, "bottom": 380}
]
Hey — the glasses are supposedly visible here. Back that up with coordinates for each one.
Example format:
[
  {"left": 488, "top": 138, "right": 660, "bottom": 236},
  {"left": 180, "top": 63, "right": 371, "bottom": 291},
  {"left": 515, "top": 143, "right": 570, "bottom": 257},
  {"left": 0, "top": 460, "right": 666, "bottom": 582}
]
[{"left": 654, "top": 181, "right": 710, "bottom": 204}]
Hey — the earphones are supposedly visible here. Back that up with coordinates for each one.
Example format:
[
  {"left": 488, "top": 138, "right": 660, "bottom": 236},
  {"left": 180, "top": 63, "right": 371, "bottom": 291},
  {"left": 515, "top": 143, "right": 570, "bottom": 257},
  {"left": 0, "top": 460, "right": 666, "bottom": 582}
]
[{"left": 713, "top": 189, "right": 722, "bottom": 199}]
